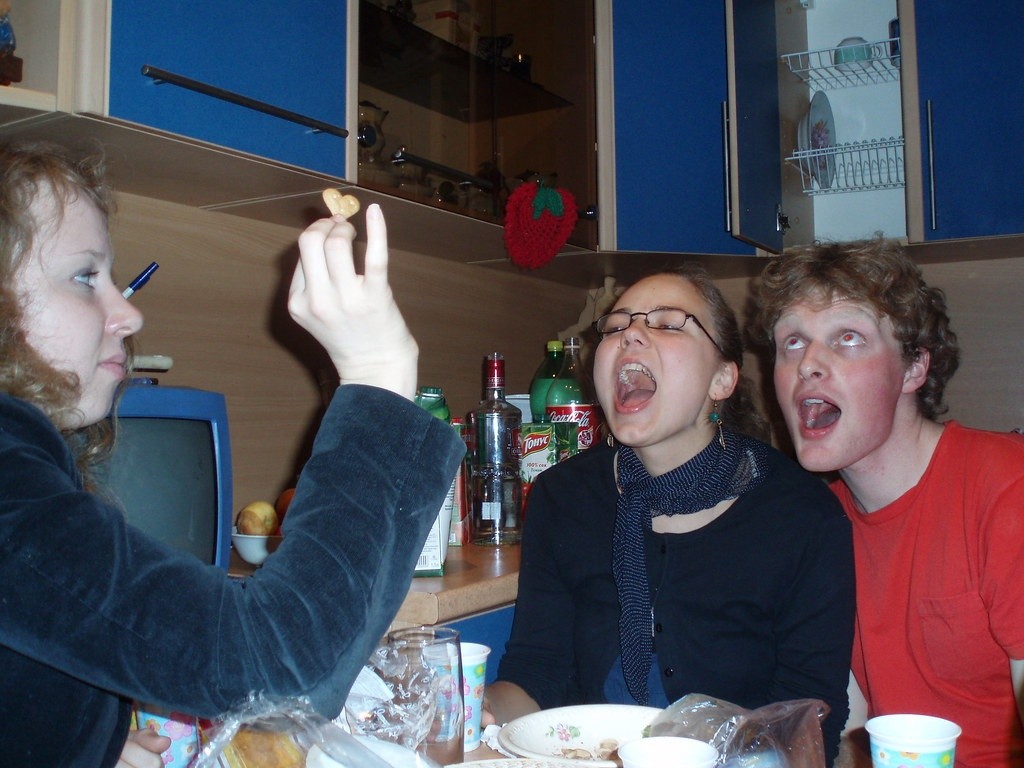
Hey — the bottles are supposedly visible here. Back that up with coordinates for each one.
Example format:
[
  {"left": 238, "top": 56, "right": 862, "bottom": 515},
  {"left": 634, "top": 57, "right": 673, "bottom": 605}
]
[
  {"left": 413, "top": 337, "right": 601, "bottom": 546},
  {"left": 358, "top": 99, "right": 427, "bottom": 198}
]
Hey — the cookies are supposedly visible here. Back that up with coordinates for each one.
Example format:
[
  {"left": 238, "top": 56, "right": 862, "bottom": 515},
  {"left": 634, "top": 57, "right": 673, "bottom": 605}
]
[{"left": 322, "top": 188, "right": 360, "bottom": 219}]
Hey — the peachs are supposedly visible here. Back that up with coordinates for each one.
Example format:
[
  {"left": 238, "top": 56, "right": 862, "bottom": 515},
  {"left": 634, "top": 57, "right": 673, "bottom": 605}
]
[{"left": 237, "top": 489, "right": 298, "bottom": 540}]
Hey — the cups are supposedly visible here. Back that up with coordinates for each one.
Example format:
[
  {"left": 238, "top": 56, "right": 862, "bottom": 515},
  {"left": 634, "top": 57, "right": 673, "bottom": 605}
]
[
  {"left": 423, "top": 642, "right": 491, "bottom": 752},
  {"left": 618, "top": 737, "right": 719, "bottom": 768},
  {"left": 834, "top": 37, "right": 882, "bottom": 72},
  {"left": 387, "top": 625, "right": 465, "bottom": 766},
  {"left": 888, "top": 16, "right": 901, "bottom": 68},
  {"left": 863, "top": 714, "right": 961, "bottom": 767}
]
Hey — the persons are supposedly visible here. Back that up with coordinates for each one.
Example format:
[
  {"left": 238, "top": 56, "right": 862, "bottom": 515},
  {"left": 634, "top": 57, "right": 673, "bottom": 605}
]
[
  {"left": 0, "top": 141, "right": 468, "bottom": 768},
  {"left": 743, "top": 241, "right": 1024, "bottom": 768},
  {"left": 422, "top": 268, "right": 854, "bottom": 768}
]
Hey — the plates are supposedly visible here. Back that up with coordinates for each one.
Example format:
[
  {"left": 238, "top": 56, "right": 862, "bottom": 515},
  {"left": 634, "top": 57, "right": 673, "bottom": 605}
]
[
  {"left": 796, "top": 90, "right": 837, "bottom": 189},
  {"left": 496, "top": 703, "right": 664, "bottom": 763}
]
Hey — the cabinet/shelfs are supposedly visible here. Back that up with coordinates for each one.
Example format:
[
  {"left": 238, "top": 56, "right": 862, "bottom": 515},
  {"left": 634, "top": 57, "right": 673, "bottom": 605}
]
[
  {"left": 76, "top": 0, "right": 359, "bottom": 205},
  {"left": 726, "top": 1, "right": 1024, "bottom": 263},
  {"left": 354, "top": 0, "right": 598, "bottom": 265},
  {"left": 604, "top": 2, "right": 769, "bottom": 260},
  {"left": 1, "top": 0, "right": 70, "bottom": 131},
  {"left": 417, "top": 607, "right": 516, "bottom": 683}
]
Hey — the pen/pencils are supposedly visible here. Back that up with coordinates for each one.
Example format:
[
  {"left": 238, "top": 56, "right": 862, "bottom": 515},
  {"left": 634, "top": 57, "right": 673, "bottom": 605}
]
[{"left": 123, "top": 261, "right": 160, "bottom": 299}]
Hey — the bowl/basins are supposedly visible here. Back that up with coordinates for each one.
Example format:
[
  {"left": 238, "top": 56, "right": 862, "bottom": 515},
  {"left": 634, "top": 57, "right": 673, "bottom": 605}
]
[{"left": 231, "top": 527, "right": 282, "bottom": 565}]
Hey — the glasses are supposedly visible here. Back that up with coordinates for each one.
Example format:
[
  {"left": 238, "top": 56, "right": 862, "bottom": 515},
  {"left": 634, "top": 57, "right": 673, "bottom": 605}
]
[{"left": 596, "top": 308, "right": 730, "bottom": 364}]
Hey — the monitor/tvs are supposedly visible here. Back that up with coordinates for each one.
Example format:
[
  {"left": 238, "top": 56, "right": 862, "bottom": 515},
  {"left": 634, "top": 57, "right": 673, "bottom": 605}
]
[{"left": 65, "top": 380, "right": 234, "bottom": 573}]
